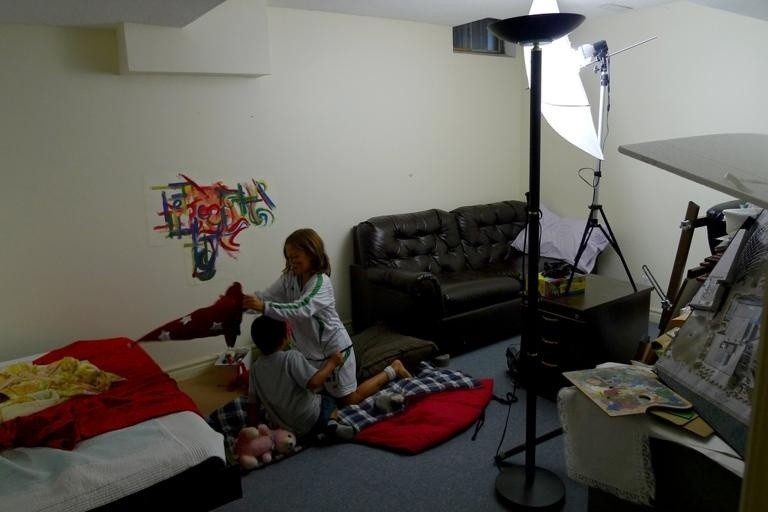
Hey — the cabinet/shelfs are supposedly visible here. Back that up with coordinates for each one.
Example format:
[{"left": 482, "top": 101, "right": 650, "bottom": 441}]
[{"left": 529, "top": 270, "right": 655, "bottom": 404}]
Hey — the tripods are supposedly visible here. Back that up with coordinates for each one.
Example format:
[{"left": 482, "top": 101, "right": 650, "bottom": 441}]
[{"left": 565, "top": 62, "right": 637, "bottom": 297}]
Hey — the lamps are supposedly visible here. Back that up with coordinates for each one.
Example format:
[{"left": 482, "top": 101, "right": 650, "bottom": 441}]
[{"left": 521, "top": 0, "right": 637, "bottom": 304}]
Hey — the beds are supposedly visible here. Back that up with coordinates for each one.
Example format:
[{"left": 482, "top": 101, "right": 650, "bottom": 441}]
[{"left": 0, "top": 335, "right": 244, "bottom": 510}]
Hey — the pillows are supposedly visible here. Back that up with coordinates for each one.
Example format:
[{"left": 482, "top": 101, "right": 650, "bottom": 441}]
[{"left": 511, "top": 199, "right": 615, "bottom": 275}]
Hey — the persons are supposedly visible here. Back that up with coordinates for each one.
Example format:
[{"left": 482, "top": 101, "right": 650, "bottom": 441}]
[
  {"left": 246, "top": 314, "right": 355, "bottom": 439},
  {"left": 218, "top": 228, "right": 413, "bottom": 407}
]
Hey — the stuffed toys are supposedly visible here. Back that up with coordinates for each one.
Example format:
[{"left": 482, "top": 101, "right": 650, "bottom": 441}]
[{"left": 234, "top": 424, "right": 297, "bottom": 470}]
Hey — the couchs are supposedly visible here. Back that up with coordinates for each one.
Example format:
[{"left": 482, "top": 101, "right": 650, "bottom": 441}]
[{"left": 345, "top": 199, "right": 600, "bottom": 359}]
